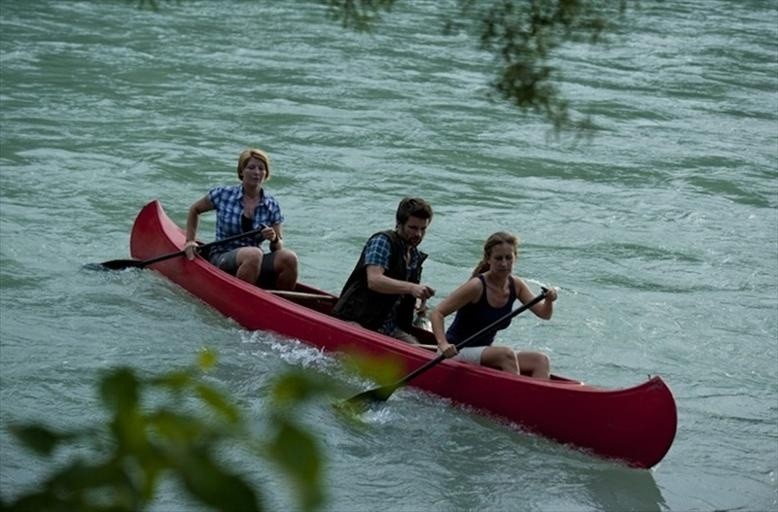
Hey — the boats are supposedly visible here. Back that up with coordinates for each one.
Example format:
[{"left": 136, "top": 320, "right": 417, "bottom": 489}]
[{"left": 129, "top": 198, "right": 680, "bottom": 472}]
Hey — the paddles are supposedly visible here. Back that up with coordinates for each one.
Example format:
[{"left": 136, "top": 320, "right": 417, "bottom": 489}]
[
  {"left": 100, "top": 222, "right": 279, "bottom": 271},
  {"left": 345, "top": 287, "right": 552, "bottom": 404}
]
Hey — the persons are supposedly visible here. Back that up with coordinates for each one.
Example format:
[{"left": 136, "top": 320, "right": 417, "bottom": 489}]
[
  {"left": 334, "top": 198, "right": 434, "bottom": 344},
  {"left": 430, "top": 234, "right": 557, "bottom": 380},
  {"left": 186, "top": 149, "right": 298, "bottom": 292}
]
